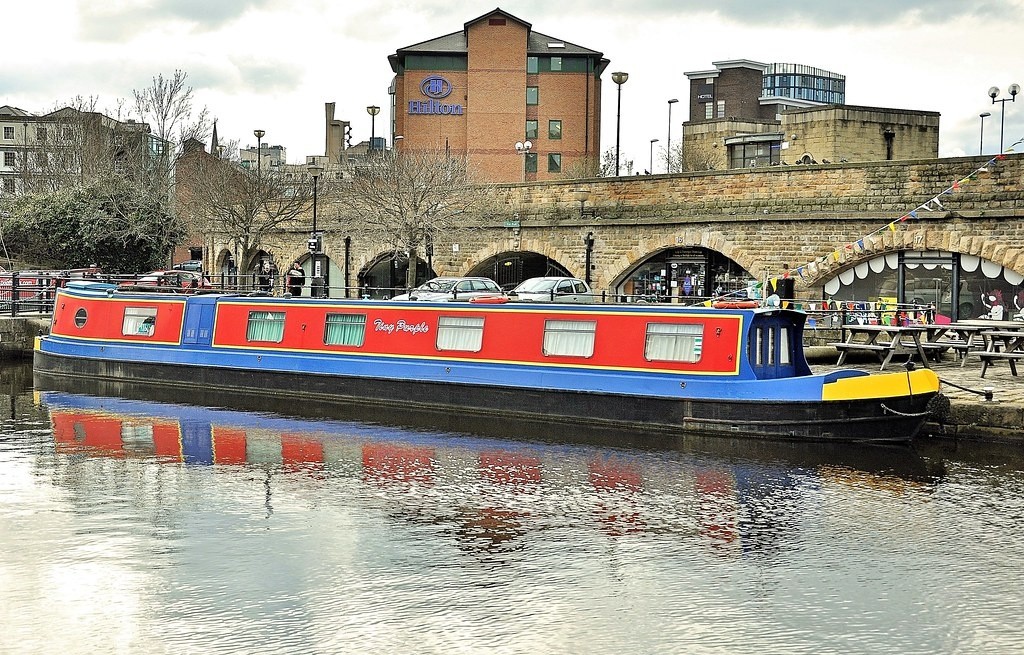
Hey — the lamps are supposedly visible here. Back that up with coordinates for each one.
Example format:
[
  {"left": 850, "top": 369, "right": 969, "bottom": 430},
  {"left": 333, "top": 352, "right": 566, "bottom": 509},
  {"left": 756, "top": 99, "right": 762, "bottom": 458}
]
[
  {"left": 712, "top": 142, "right": 718, "bottom": 148},
  {"left": 791, "top": 133, "right": 796, "bottom": 140}
]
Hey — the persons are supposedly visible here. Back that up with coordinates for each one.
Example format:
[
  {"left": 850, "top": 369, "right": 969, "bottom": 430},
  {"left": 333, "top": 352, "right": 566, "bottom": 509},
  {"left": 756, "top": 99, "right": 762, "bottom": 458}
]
[{"left": 287, "top": 261, "right": 305, "bottom": 298}]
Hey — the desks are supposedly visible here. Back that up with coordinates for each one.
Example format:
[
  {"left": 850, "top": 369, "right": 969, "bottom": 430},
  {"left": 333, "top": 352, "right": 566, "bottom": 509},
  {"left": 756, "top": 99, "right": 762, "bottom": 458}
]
[
  {"left": 956, "top": 318, "right": 1024, "bottom": 353},
  {"left": 978, "top": 330, "right": 1024, "bottom": 380},
  {"left": 907, "top": 323, "right": 998, "bottom": 368},
  {"left": 834, "top": 325, "right": 931, "bottom": 371},
  {"left": 947, "top": 322, "right": 1021, "bottom": 364}
]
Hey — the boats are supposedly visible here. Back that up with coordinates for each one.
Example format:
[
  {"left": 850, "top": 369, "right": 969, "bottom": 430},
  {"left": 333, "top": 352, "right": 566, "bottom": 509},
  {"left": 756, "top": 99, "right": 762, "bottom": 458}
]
[{"left": 32, "top": 282, "right": 945, "bottom": 444}]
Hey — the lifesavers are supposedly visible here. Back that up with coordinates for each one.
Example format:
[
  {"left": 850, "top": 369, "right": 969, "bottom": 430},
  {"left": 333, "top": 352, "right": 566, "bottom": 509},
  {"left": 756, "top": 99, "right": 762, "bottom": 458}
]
[
  {"left": 712, "top": 299, "right": 758, "bottom": 308},
  {"left": 469, "top": 295, "right": 509, "bottom": 303}
]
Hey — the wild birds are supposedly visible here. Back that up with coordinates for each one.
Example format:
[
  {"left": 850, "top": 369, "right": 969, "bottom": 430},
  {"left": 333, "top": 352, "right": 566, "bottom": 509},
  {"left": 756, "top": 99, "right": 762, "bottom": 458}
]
[
  {"left": 770, "top": 158, "right": 848, "bottom": 165},
  {"left": 636, "top": 172, "right": 639, "bottom": 176},
  {"left": 644, "top": 169, "right": 650, "bottom": 175}
]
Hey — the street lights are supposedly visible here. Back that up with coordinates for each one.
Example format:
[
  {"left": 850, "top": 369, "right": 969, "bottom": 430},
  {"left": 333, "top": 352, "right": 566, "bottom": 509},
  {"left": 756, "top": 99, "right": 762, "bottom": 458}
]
[
  {"left": 987, "top": 83, "right": 1021, "bottom": 154},
  {"left": 367, "top": 105, "right": 381, "bottom": 162},
  {"left": 667, "top": 99, "right": 679, "bottom": 172},
  {"left": 344, "top": 236, "right": 354, "bottom": 297},
  {"left": 253, "top": 129, "right": 265, "bottom": 197},
  {"left": 583, "top": 232, "right": 595, "bottom": 286},
  {"left": 216, "top": 144, "right": 226, "bottom": 161},
  {"left": 650, "top": 139, "right": 659, "bottom": 175},
  {"left": 305, "top": 164, "right": 324, "bottom": 277},
  {"left": 612, "top": 71, "right": 628, "bottom": 176},
  {"left": 516, "top": 141, "right": 533, "bottom": 183},
  {"left": 978, "top": 112, "right": 990, "bottom": 156}
]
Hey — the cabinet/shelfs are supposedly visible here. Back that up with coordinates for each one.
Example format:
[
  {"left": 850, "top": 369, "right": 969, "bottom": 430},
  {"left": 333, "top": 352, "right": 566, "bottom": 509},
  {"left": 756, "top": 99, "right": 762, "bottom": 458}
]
[{"left": 633, "top": 263, "right": 755, "bottom": 304}]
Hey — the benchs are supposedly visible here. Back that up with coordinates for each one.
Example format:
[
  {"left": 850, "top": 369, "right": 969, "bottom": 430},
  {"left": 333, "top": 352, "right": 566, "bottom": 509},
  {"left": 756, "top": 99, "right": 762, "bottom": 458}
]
[{"left": 829, "top": 337, "right": 1024, "bottom": 359}]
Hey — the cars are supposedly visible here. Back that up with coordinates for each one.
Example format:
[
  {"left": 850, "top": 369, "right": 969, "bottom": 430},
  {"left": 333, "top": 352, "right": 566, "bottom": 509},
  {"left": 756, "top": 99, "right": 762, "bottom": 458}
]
[
  {"left": 118, "top": 269, "right": 212, "bottom": 297},
  {"left": 173, "top": 259, "right": 203, "bottom": 272},
  {"left": 508, "top": 276, "right": 594, "bottom": 303},
  {"left": 391, "top": 276, "right": 528, "bottom": 302}
]
